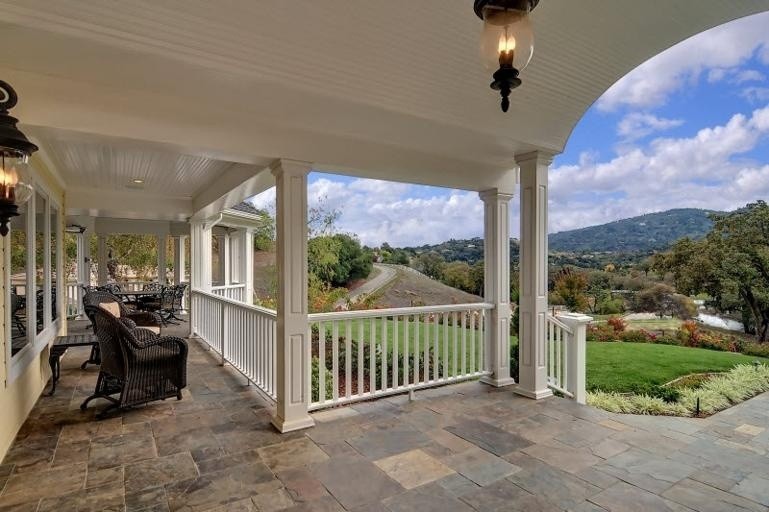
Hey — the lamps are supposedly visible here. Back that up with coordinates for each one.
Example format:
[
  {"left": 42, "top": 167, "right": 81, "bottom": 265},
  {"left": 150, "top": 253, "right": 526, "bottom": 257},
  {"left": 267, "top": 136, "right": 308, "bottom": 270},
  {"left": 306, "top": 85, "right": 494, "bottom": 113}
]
[
  {"left": 0, "top": 80, "right": 39, "bottom": 235},
  {"left": 473, "top": 0, "right": 539, "bottom": 112}
]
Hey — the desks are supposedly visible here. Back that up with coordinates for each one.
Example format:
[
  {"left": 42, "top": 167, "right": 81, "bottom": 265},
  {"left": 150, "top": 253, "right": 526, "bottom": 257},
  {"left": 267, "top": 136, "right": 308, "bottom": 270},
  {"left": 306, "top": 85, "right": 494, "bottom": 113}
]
[{"left": 49, "top": 335, "right": 99, "bottom": 395}]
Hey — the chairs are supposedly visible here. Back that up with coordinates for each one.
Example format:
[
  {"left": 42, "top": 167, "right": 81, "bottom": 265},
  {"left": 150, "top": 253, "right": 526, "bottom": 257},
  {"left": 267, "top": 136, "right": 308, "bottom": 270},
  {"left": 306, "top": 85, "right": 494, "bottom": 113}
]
[
  {"left": 80, "top": 282, "right": 189, "bottom": 420},
  {"left": 12, "top": 281, "right": 57, "bottom": 335}
]
